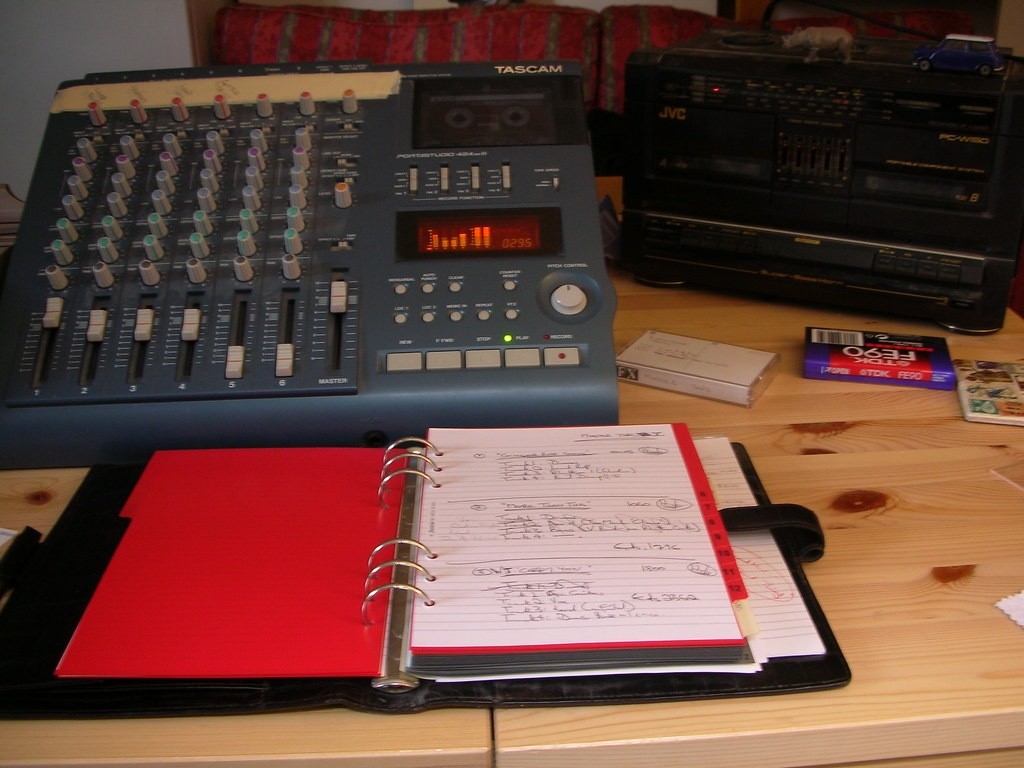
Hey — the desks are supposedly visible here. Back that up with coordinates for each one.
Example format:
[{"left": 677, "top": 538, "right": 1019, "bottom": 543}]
[{"left": 0, "top": 178, "right": 1024, "bottom": 768}]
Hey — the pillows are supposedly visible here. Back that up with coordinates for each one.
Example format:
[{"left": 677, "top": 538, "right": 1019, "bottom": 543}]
[
  {"left": 596, "top": 7, "right": 972, "bottom": 116},
  {"left": 213, "top": 4, "right": 602, "bottom": 114}
]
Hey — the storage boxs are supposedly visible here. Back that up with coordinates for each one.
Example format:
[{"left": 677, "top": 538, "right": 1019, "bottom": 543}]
[
  {"left": 613, "top": 331, "right": 783, "bottom": 406},
  {"left": 799, "top": 326, "right": 958, "bottom": 390}
]
